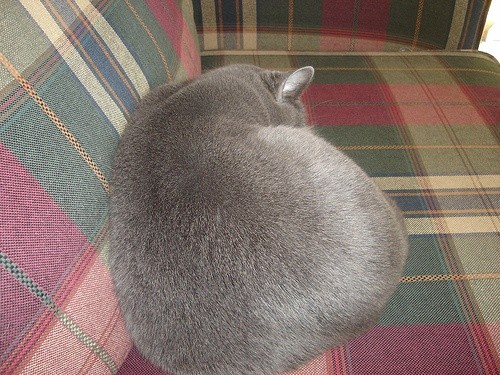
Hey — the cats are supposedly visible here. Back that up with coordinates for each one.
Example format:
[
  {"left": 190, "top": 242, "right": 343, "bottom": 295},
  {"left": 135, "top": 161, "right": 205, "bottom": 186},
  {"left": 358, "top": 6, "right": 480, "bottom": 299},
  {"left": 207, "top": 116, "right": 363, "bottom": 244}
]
[{"left": 106, "top": 62, "right": 413, "bottom": 375}]
